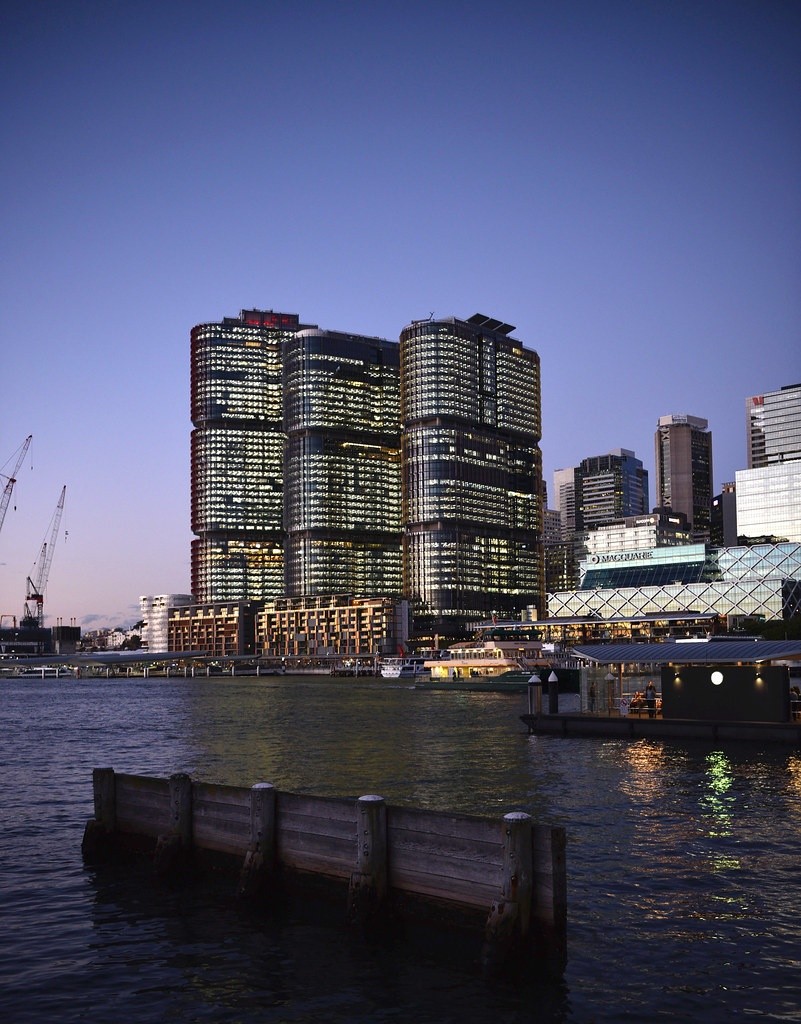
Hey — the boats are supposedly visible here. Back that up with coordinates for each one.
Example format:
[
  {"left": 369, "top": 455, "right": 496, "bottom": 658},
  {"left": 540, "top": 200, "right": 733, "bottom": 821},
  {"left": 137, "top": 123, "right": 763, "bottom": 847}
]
[
  {"left": 6, "top": 667, "right": 71, "bottom": 679},
  {"left": 380, "top": 657, "right": 448, "bottom": 678},
  {"left": 414, "top": 642, "right": 541, "bottom": 695},
  {"left": 520, "top": 642, "right": 801, "bottom": 744}
]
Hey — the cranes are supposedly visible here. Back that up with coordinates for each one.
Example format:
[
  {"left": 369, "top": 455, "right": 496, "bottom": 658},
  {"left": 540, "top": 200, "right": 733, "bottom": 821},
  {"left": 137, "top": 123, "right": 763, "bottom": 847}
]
[
  {"left": 22, "top": 484, "right": 69, "bottom": 628},
  {"left": 0, "top": 434, "right": 33, "bottom": 531}
]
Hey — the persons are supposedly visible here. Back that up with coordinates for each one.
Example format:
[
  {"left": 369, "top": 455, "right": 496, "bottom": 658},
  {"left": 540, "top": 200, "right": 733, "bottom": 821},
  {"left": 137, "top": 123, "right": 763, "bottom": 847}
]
[
  {"left": 646, "top": 681, "right": 656, "bottom": 718},
  {"left": 630, "top": 691, "right": 641, "bottom": 714},
  {"left": 590, "top": 683, "right": 596, "bottom": 713}
]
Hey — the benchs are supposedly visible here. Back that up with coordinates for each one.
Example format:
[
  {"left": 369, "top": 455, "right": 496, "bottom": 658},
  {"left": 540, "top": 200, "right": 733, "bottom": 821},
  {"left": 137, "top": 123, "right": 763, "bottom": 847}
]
[{"left": 607, "top": 699, "right": 661, "bottom": 718}]
